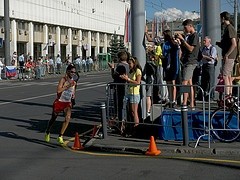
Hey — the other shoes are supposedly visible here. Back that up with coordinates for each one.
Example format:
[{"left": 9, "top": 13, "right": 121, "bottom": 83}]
[
  {"left": 182, "top": 103, "right": 188, "bottom": 108},
  {"left": 162, "top": 101, "right": 170, "bottom": 107},
  {"left": 158, "top": 99, "right": 166, "bottom": 104},
  {"left": 170, "top": 102, "right": 176, "bottom": 108}
]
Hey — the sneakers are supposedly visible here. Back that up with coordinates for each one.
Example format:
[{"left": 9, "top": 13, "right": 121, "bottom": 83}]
[
  {"left": 45, "top": 132, "right": 50, "bottom": 142},
  {"left": 57, "top": 137, "right": 64, "bottom": 144}
]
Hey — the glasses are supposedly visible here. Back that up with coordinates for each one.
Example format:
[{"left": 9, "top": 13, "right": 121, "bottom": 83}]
[{"left": 69, "top": 70, "right": 77, "bottom": 74}]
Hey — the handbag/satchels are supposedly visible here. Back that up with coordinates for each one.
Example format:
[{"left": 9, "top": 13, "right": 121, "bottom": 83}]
[{"left": 197, "top": 49, "right": 203, "bottom": 62}]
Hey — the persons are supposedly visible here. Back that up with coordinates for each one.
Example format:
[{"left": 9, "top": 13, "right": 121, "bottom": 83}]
[
  {"left": 107, "top": 12, "right": 238, "bottom": 127},
  {"left": 45, "top": 64, "right": 77, "bottom": 146},
  {"left": 12, "top": 51, "right": 61, "bottom": 80},
  {"left": 65, "top": 56, "right": 94, "bottom": 73}
]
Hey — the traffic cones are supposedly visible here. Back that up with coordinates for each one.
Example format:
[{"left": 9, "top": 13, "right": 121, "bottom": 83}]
[
  {"left": 107, "top": 116, "right": 115, "bottom": 126},
  {"left": 71, "top": 133, "right": 85, "bottom": 150},
  {"left": 144, "top": 135, "right": 162, "bottom": 156},
  {"left": 90, "top": 125, "right": 98, "bottom": 138}
]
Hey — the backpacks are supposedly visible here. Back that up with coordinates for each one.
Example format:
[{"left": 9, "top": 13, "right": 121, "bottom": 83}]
[{"left": 202, "top": 46, "right": 218, "bottom": 66}]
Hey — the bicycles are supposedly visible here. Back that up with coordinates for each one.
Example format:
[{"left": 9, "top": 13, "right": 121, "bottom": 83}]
[{"left": 210, "top": 92, "right": 240, "bottom": 144}]
